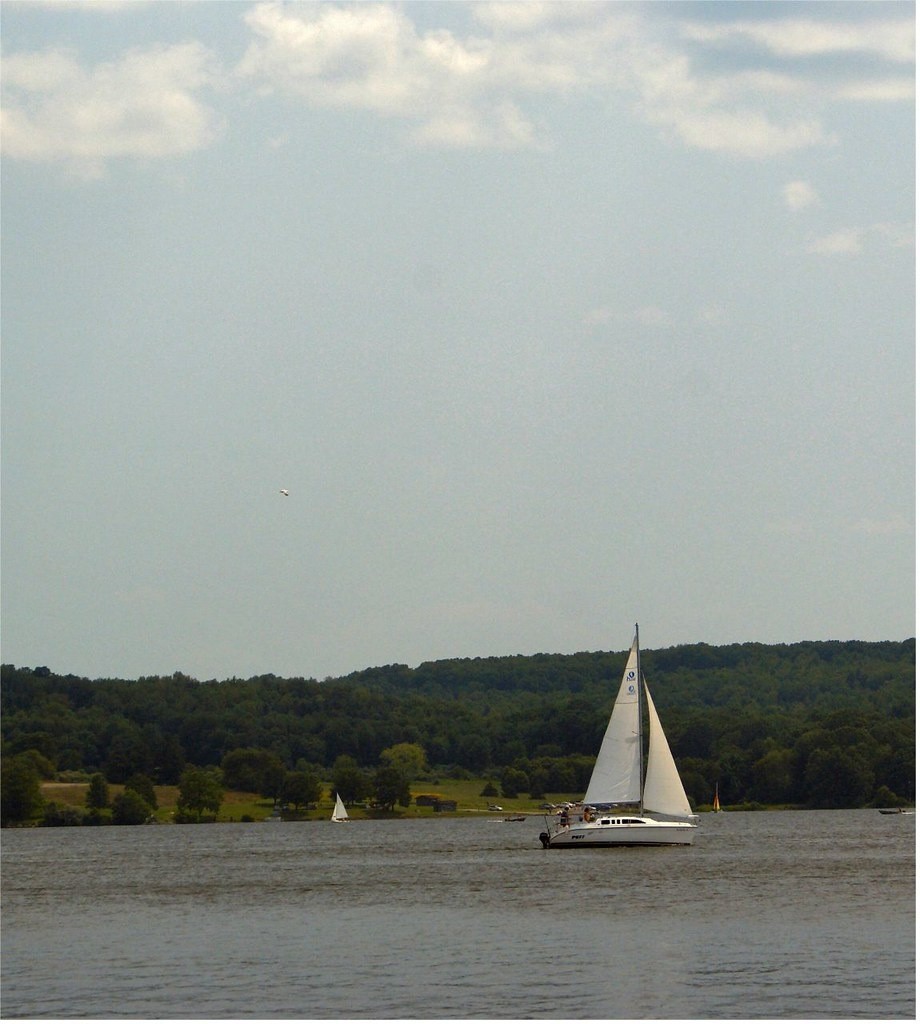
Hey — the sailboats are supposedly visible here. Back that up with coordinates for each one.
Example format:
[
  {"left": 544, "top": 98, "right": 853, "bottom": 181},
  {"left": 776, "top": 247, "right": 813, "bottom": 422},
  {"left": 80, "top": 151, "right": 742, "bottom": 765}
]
[
  {"left": 330, "top": 792, "right": 351, "bottom": 823},
  {"left": 539, "top": 623, "right": 701, "bottom": 847},
  {"left": 709, "top": 781, "right": 723, "bottom": 813}
]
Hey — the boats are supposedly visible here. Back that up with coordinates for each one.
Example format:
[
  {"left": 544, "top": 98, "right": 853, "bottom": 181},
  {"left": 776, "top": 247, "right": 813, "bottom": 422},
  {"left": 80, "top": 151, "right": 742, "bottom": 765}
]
[
  {"left": 878, "top": 808, "right": 906, "bottom": 814},
  {"left": 504, "top": 815, "right": 528, "bottom": 821}
]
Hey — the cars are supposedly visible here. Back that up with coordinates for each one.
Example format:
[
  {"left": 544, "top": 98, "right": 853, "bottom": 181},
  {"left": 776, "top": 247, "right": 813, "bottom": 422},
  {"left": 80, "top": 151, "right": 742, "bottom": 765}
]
[
  {"left": 488, "top": 805, "right": 503, "bottom": 811},
  {"left": 540, "top": 803, "right": 556, "bottom": 809},
  {"left": 555, "top": 800, "right": 618, "bottom": 810}
]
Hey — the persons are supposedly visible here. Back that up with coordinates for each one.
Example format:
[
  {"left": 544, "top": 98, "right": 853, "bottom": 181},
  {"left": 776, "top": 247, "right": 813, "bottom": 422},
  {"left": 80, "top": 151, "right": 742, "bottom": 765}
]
[
  {"left": 560, "top": 807, "right": 570, "bottom": 828},
  {"left": 898, "top": 807, "right": 901, "bottom": 812},
  {"left": 282, "top": 800, "right": 288, "bottom": 812},
  {"left": 273, "top": 804, "right": 279, "bottom": 816},
  {"left": 583, "top": 808, "right": 590, "bottom": 823}
]
